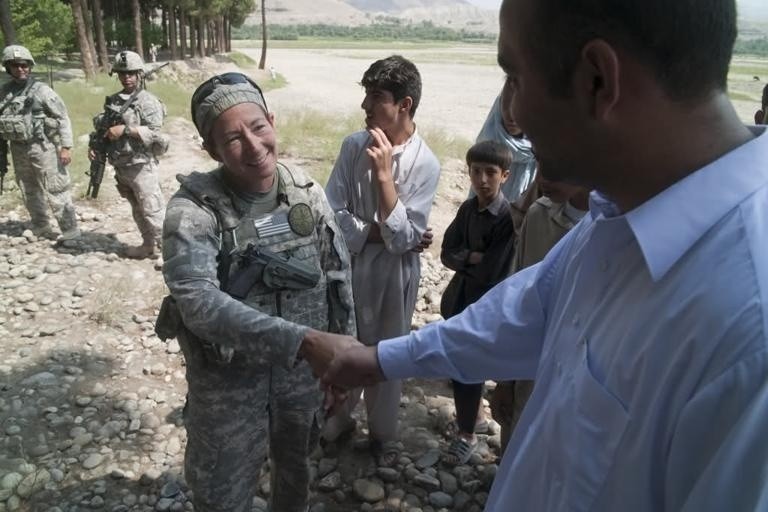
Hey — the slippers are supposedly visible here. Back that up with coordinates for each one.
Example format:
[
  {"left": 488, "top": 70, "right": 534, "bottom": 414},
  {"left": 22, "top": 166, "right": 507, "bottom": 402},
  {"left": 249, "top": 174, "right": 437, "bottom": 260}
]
[{"left": 442, "top": 439, "right": 478, "bottom": 465}]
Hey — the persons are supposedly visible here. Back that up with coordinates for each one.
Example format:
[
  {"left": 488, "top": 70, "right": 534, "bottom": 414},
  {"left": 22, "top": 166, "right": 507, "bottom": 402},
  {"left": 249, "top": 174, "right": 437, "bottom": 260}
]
[
  {"left": 318, "top": 53, "right": 439, "bottom": 467},
  {"left": 149, "top": 43, "right": 158, "bottom": 62},
  {"left": 162, "top": 72, "right": 358, "bottom": 512},
  {"left": 88, "top": 51, "right": 170, "bottom": 270},
  {"left": 318, "top": 0, "right": 767, "bottom": 510},
  {"left": 439, "top": 77, "right": 592, "bottom": 466},
  {"left": 0, "top": 45, "right": 82, "bottom": 241}
]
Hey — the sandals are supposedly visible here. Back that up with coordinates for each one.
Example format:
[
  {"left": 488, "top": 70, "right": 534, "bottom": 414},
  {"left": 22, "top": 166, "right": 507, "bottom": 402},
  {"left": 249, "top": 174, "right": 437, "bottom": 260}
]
[{"left": 372, "top": 440, "right": 400, "bottom": 467}]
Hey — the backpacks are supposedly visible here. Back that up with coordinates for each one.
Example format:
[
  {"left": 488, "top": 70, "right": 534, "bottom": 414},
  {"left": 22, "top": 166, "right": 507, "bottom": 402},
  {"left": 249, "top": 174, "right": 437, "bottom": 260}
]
[{"left": 106, "top": 105, "right": 140, "bottom": 156}]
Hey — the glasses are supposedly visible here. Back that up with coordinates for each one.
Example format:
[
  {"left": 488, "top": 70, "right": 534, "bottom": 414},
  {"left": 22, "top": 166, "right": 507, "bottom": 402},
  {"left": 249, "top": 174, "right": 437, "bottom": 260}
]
[
  {"left": 7, "top": 63, "right": 30, "bottom": 68},
  {"left": 191, "top": 73, "right": 268, "bottom": 129}
]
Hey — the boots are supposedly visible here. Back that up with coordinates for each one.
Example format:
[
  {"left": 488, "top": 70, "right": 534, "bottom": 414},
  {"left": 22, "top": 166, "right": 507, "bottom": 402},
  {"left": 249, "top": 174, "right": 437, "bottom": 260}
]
[
  {"left": 22, "top": 224, "right": 51, "bottom": 237},
  {"left": 56, "top": 226, "right": 81, "bottom": 241},
  {"left": 126, "top": 238, "right": 164, "bottom": 270}
]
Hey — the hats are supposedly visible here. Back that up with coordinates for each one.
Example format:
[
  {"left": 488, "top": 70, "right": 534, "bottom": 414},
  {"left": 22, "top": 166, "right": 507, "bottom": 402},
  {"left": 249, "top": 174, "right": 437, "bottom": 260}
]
[{"left": 191, "top": 76, "right": 268, "bottom": 142}]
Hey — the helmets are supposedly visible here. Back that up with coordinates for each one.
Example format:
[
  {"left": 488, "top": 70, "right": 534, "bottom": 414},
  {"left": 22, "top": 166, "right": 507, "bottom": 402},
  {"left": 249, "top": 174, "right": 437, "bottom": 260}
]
[
  {"left": 111, "top": 50, "right": 144, "bottom": 73},
  {"left": 1, "top": 44, "right": 36, "bottom": 67}
]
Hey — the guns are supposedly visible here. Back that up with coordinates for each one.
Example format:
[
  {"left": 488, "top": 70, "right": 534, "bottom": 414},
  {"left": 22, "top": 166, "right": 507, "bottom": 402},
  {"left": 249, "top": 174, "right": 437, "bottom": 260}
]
[
  {"left": 226, "top": 243, "right": 320, "bottom": 299},
  {"left": 1, "top": 136, "right": 10, "bottom": 195},
  {"left": 87, "top": 96, "right": 121, "bottom": 198}
]
